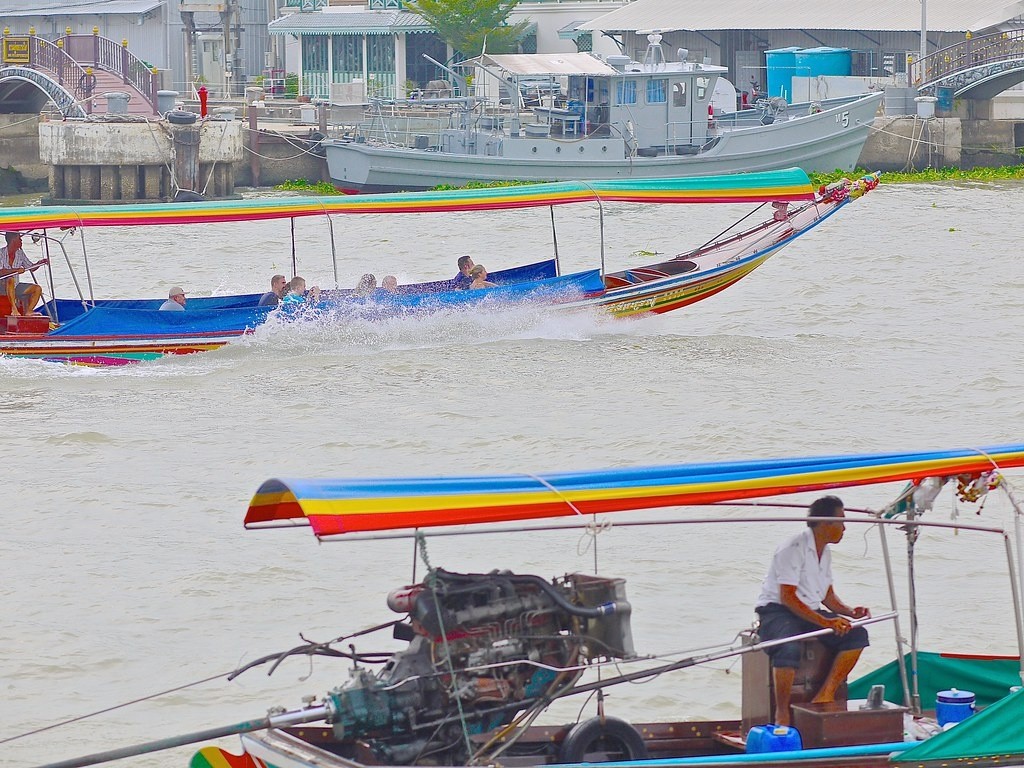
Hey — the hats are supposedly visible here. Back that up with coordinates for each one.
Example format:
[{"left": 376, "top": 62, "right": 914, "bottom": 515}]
[{"left": 169, "top": 287, "right": 191, "bottom": 296}]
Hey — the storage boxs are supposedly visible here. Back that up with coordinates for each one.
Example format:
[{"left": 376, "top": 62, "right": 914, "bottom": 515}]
[{"left": 790, "top": 698, "right": 911, "bottom": 749}]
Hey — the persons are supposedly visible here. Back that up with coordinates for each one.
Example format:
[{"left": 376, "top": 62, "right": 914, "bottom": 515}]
[
  {"left": 258, "top": 275, "right": 321, "bottom": 306},
  {"left": 447, "top": 256, "right": 499, "bottom": 290},
  {"left": 0, "top": 232, "right": 50, "bottom": 316},
  {"left": 351, "top": 273, "right": 397, "bottom": 298},
  {"left": 754, "top": 496, "right": 872, "bottom": 726},
  {"left": 159, "top": 287, "right": 190, "bottom": 310}
]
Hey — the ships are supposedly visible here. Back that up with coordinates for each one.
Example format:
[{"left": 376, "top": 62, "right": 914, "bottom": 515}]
[{"left": 319, "top": 27, "right": 885, "bottom": 194}]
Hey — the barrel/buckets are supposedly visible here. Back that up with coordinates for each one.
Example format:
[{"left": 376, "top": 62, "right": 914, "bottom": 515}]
[
  {"left": 935, "top": 689, "right": 976, "bottom": 725},
  {"left": 744, "top": 724, "right": 802, "bottom": 754}
]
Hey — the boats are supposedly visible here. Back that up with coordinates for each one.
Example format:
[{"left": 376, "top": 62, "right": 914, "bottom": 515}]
[
  {"left": 0, "top": 165, "right": 884, "bottom": 369},
  {"left": 35, "top": 444, "right": 1024, "bottom": 768}
]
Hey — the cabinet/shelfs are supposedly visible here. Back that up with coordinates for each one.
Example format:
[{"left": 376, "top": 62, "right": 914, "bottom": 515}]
[{"left": 739, "top": 632, "right": 847, "bottom": 741}]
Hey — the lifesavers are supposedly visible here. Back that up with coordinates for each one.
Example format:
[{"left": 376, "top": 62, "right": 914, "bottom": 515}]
[{"left": 551, "top": 711, "right": 659, "bottom": 766}]
